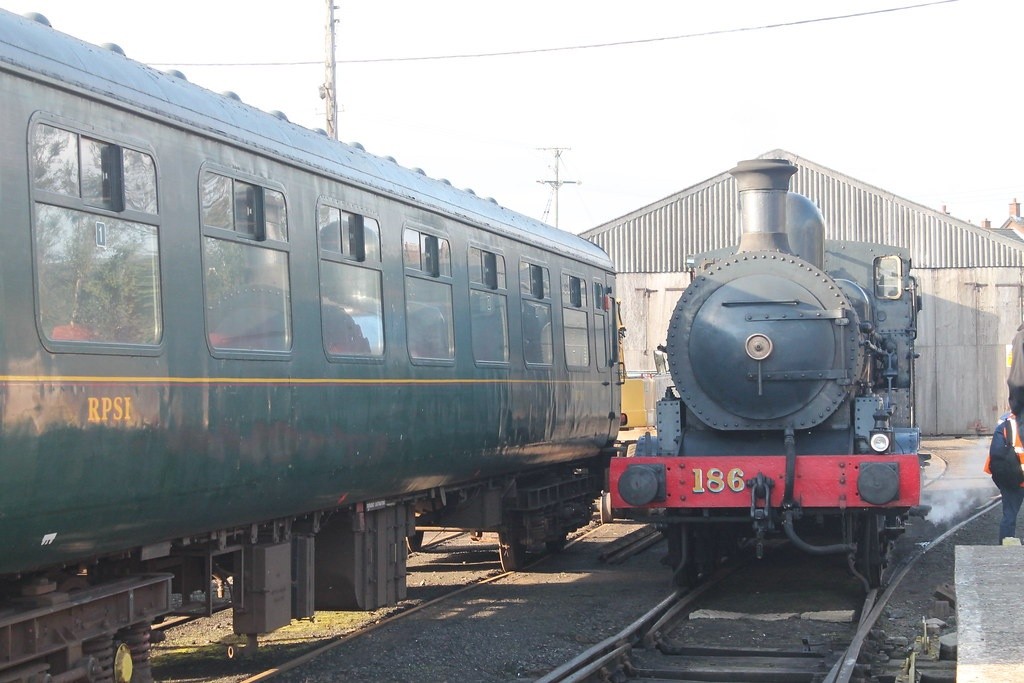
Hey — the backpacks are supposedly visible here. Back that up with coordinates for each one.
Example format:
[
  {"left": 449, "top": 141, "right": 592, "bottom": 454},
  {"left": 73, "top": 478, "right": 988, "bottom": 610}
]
[{"left": 989, "top": 421, "right": 1024, "bottom": 489}]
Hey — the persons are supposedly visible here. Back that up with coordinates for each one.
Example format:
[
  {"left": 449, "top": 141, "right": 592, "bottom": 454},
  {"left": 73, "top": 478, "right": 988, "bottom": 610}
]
[{"left": 989, "top": 322, "right": 1024, "bottom": 545}]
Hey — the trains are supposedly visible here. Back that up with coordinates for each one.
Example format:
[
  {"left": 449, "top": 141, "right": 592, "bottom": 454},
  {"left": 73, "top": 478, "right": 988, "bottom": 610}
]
[
  {"left": 0, "top": 4, "right": 618, "bottom": 682},
  {"left": 600, "top": 157, "right": 932, "bottom": 584}
]
